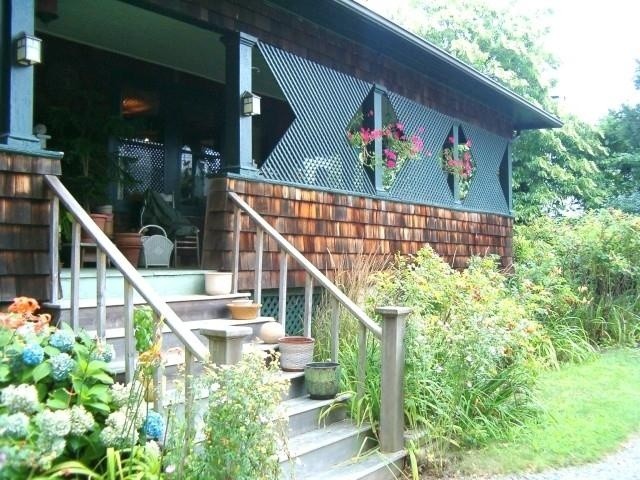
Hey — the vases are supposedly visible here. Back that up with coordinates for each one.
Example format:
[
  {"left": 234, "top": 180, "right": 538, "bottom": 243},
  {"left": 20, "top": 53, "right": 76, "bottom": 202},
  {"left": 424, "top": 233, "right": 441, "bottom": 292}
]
[
  {"left": 117, "top": 242, "right": 143, "bottom": 269},
  {"left": 303, "top": 362, "right": 340, "bottom": 400},
  {"left": 227, "top": 299, "right": 262, "bottom": 319},
  {"left": 278, "top": 336, "right": 315, "bottom": 372},
  {"left": 114, "top": 232, "right": 143, "bottom": 246},
  {"left": 204, "top": 272, "right": 233, "bottom": 294}
]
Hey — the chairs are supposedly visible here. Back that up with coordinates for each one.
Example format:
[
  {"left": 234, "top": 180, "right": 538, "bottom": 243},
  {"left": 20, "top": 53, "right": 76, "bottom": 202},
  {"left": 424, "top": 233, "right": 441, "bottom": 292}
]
[{"left": 161, "top": 188, "right": 201, "bottom": 267}]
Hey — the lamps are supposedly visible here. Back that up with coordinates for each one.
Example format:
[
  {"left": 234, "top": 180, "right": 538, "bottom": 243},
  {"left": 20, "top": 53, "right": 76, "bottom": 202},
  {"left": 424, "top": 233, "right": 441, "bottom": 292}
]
[
  {"left": 244, "top": 90, "right": 263, "bottom": 116},
  {"left": 15, "top": 31, "right": 44, "bottom": 66}
]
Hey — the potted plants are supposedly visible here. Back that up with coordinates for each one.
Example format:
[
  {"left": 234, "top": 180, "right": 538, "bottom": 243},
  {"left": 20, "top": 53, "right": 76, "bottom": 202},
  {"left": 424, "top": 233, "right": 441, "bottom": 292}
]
[{"left": 53, "top": 137, "right": 143, "bottom": 244}]
